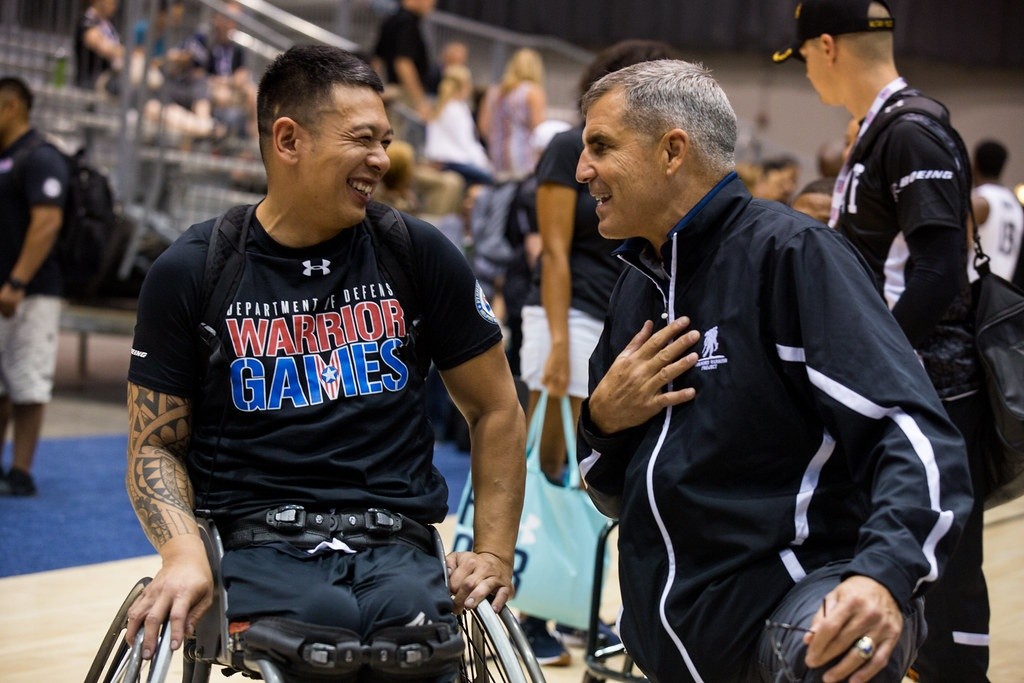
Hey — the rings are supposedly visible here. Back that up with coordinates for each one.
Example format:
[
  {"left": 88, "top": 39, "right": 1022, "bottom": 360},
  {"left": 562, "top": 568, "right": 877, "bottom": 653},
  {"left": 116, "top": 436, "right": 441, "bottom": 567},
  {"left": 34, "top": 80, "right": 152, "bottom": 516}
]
[{"left": 854, "top": 635, "right": 876, "bottom": 659}]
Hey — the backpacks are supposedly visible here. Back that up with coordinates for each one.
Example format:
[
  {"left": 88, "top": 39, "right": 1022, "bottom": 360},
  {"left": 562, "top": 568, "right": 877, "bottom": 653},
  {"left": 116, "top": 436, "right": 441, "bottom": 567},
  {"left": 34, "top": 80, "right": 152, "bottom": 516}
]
[{"left": 11, "top": 139, "right": 114, "bottom": 284}]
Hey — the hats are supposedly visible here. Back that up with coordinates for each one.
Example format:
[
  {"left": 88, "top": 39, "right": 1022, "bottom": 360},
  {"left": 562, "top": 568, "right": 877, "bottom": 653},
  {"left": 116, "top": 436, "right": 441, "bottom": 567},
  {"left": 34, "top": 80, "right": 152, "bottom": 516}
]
[{"left": 772, "top": 0, "right": 896, "bottom": 64}]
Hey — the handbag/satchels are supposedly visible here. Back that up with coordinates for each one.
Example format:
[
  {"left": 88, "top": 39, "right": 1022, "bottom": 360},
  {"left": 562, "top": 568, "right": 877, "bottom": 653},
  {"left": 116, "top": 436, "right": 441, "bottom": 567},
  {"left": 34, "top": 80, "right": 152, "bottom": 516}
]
[
  {"left": 920, "top": 272, "right": 1023, "bottom": 513},
  {"left": 450, "top": 388, "right": 613, "bottom": 632}
]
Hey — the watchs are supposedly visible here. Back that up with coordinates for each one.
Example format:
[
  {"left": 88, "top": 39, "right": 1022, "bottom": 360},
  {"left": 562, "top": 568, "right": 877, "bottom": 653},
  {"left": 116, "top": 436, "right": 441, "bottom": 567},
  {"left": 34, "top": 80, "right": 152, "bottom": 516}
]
[{"left": 6, "top": 275, "right": 28, "bottom": 292}]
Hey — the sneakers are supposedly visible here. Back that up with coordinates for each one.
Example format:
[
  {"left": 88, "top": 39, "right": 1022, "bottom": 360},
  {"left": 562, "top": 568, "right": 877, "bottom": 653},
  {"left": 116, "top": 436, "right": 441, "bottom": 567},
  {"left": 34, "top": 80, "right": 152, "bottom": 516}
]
[
  {"left": 552, "top": 618, "right": 625, "bottom": 657},
  {"left": 517, "top": 617, "right": 572, "bottom": 667}
]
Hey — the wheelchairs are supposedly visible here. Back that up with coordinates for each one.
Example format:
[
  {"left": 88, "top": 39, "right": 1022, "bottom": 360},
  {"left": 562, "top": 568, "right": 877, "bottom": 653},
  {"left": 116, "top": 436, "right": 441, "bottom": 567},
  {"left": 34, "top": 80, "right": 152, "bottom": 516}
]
[{"left": 83, "top": 507, "right": 548, "bottom": 683}]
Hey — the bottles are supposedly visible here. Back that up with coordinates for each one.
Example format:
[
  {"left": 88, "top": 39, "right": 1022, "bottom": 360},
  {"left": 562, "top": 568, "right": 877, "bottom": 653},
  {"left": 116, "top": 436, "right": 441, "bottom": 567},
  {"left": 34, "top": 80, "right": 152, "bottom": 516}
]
[{"left": 53, "top": 47, "right": 69, "bottom": 89}]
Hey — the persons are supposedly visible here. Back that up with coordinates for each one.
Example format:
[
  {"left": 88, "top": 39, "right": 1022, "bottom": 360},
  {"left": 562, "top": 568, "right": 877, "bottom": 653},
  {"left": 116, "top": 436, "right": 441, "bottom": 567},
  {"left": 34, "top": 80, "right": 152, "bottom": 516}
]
[
  {"left": 0, "top": 76, "right": 72, "bottom": 501},
  {"left": 73, "top": 0, "right": 1024, "bottom": 457},
  {"left": 509, "top": 40, "right": 674, "bottom": 666},
  {"left": 126, "top": 44, "right": 528, "bottom": 683},
  {"left": 576, "top": 58, "right": 975, "bottom": 683}
]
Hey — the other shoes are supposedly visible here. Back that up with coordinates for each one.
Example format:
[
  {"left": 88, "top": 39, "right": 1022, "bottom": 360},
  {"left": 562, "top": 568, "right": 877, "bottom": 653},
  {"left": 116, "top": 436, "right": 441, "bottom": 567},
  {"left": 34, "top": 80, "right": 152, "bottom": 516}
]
[{"left": 0, "top": 467, "right": 36, "bottom": 497}]
